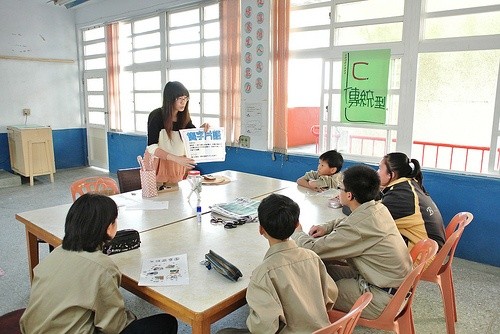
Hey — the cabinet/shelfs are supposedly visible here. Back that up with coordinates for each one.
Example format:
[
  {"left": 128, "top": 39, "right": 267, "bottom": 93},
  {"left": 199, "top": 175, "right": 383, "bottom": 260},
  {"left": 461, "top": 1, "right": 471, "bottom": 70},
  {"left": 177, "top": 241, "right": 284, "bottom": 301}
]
[{"left": 7, "top": 126, "right": 56, "bottom": 185}]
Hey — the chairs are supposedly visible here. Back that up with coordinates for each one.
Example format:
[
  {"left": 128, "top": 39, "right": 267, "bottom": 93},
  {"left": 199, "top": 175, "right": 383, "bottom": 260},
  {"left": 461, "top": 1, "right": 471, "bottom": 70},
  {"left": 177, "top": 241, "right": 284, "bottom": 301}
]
[
  {"left": 420, "top": 212, "right": 473, "bottom": 334},
  {"left": 71, "top": 167, "right": 142, "bottom": 201},
  {"left": 330, "top": 239, "right": 439, "bottom": 334},
  {"left": 313, "top": 292, "right": 373, "bottom": 334}
]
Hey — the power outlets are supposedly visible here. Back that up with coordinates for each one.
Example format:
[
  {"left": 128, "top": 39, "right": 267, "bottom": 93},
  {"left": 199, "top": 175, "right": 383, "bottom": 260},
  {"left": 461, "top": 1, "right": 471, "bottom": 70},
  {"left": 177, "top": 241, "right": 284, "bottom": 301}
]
[{"left": 22, "top": 109, "right": 29, "bottom": 116}]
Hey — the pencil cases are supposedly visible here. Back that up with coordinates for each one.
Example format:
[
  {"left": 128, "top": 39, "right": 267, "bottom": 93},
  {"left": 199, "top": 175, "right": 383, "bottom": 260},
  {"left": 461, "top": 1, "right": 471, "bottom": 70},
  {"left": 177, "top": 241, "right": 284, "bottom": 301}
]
[
  {"left": 103, "top": 228, "right": 140, "bottom": 254},
  {"left": 328, "top": 194, "right": 341, "bottom": 208},
  {"left": 204, "top": 249, "right": 243, "bottom": 282}
]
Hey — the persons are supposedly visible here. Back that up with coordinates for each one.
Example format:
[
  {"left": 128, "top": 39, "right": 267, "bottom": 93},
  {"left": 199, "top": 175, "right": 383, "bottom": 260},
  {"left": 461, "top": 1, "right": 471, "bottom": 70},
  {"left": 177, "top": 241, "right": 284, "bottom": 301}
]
[
  {"left": 19, "top": 193, "right": 178, "bottom": 334},
  {"left": 215, "top": 194, "right": 339, "bottom": 334},
  {"left": 297, "top": 150, "right": 343, "bottom": 192},
  {"left": 377, "top": 153, "right": 452, "bottom": 276},
  {"left": 290, "top": 164, "right": 415, "bottom": 320},
  {"left": 141, "top": 81, "right": 211, "bottom": 186}
]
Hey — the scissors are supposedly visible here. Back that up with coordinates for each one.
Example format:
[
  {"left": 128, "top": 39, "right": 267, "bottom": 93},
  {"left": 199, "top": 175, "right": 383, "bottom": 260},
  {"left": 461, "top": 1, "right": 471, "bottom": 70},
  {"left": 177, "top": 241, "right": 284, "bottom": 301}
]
[
  {"left": 223, "top": 220, "right": 239, "bottom": 228},
  {"left": 210, "top": 214, "right": 222, "bottom": 223}
]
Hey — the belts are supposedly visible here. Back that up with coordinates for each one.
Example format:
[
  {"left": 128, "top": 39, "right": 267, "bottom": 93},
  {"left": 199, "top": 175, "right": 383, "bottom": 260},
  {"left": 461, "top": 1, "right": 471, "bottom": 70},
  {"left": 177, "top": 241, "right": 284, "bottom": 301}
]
[{"left": 367, "top": 282, "right": 410, "bottom": 298}]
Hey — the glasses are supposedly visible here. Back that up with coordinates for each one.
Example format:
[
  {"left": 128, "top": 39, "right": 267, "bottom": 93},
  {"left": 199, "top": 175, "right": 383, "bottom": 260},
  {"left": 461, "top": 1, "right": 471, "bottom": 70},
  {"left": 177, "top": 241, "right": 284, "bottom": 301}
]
[
  {"left": 336, "top": 185, "right": 348, "bottom": 193},
  {"left": 175, "top": 96, "right": 190, "bottom": 102}
]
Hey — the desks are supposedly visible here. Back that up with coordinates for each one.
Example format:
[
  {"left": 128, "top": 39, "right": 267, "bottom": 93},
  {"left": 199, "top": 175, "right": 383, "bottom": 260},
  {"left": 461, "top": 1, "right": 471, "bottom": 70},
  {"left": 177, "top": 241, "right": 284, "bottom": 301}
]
[{"left": 15, "top": 169, "right": 348, "bottom": 334}]
